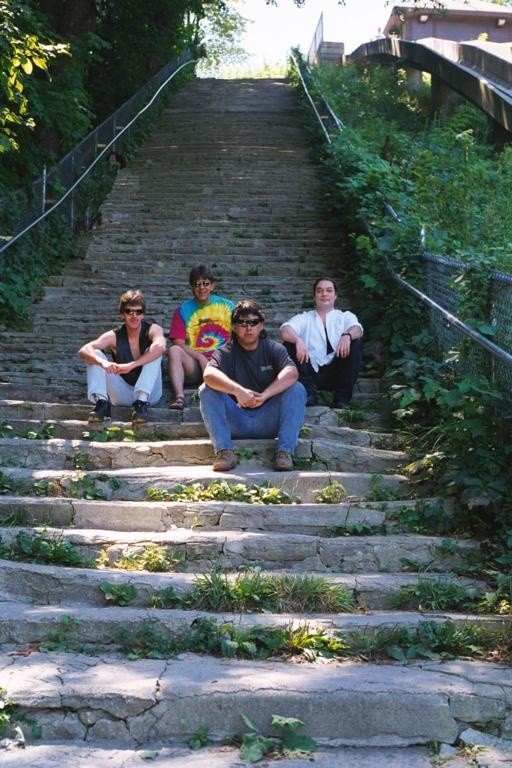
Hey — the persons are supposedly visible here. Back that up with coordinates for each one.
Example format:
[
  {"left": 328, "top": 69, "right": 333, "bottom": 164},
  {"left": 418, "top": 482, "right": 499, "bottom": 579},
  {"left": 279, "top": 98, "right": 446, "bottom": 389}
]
[
  {"left": 77, "top": 288, "right": 166, "bottom": 424},
  {"left": 197, "top": 298, "right": 308, "bottom": 472},
  {"left": 168, "top": 264, "right": 236, "bottom": 410},
  {"left": 278, "top": 275, "right": 365, "bottom": 408}
]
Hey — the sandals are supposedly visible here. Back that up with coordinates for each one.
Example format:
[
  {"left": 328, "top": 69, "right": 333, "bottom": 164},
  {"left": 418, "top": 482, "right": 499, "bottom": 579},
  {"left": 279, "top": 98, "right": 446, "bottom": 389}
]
[{"left": 169, "top": 396, "right": 186, "bottom": 410}]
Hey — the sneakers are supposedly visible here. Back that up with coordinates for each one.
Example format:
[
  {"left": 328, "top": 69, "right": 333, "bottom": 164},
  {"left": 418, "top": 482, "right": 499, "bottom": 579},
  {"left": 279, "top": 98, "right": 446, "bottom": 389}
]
[
  {"left": 213, "top": 448, "right": 239, "bottom": 471},
  {"left": 273, "top": 448, "right": 294, "bottom": 471},
  {"left": 130, "top": 399, "right": 148, "bottom": 422},
  {"left": 335, "top": 389, "right": 352, "bottom": 409},
  {"left": 306, "top": 384, "right": 320, "bottom": 406},
  {"left": 88, "top": 399, "right": 111, "bottom": 421}
]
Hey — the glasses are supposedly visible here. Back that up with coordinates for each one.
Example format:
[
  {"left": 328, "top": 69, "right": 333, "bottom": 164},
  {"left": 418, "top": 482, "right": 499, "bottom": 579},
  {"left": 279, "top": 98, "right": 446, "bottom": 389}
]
[
  {"left": 125, "top": 308, "right": 143, "bottom": 316},
  {"left": 192, "top": 281, "right": 209, "bottom": 288},
  {"left": 237, "top": 318, "right": 259, "bottom": 327}
]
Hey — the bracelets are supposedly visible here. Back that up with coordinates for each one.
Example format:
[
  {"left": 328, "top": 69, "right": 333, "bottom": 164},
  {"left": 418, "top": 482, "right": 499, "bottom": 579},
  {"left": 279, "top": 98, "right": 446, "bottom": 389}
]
[{"left": 342, "top": 331, "right": 352, "bottom": 338}]
